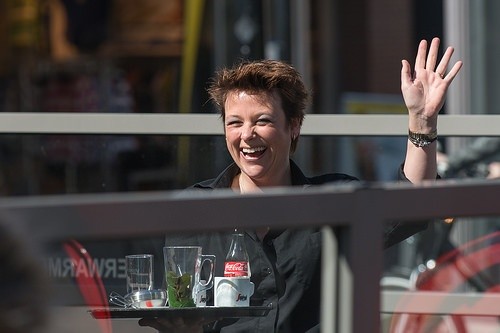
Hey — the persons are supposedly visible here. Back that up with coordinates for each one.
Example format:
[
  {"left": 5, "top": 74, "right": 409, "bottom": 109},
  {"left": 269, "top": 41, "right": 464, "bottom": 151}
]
[{"left": 137, "top": 36, "right": 463, "bottom": 333}]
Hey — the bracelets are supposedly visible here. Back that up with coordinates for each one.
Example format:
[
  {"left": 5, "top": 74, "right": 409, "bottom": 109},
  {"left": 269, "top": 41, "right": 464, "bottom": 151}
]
[{"left": 407, "top": 129, "right": 437, "bottom": 147}]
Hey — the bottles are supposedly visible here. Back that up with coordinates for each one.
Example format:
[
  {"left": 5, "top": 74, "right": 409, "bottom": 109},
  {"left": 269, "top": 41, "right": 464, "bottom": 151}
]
[{"left": 224, "top": 233, "right": 248, "bottom": 277}]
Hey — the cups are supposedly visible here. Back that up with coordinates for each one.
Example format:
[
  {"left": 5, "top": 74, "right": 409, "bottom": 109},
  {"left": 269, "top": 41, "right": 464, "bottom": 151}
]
[
  {"left": 163, "top": 246, "right": 216, "bottom": 308},
  {"left": 125, "top": 254, "right": 153, "bottom": 292},
  {"left": 195, "top": 280, "right": 207, "bottom": 307},
  {"left": 213, "top": 276, "right": 254, "bottom": 306}
]
